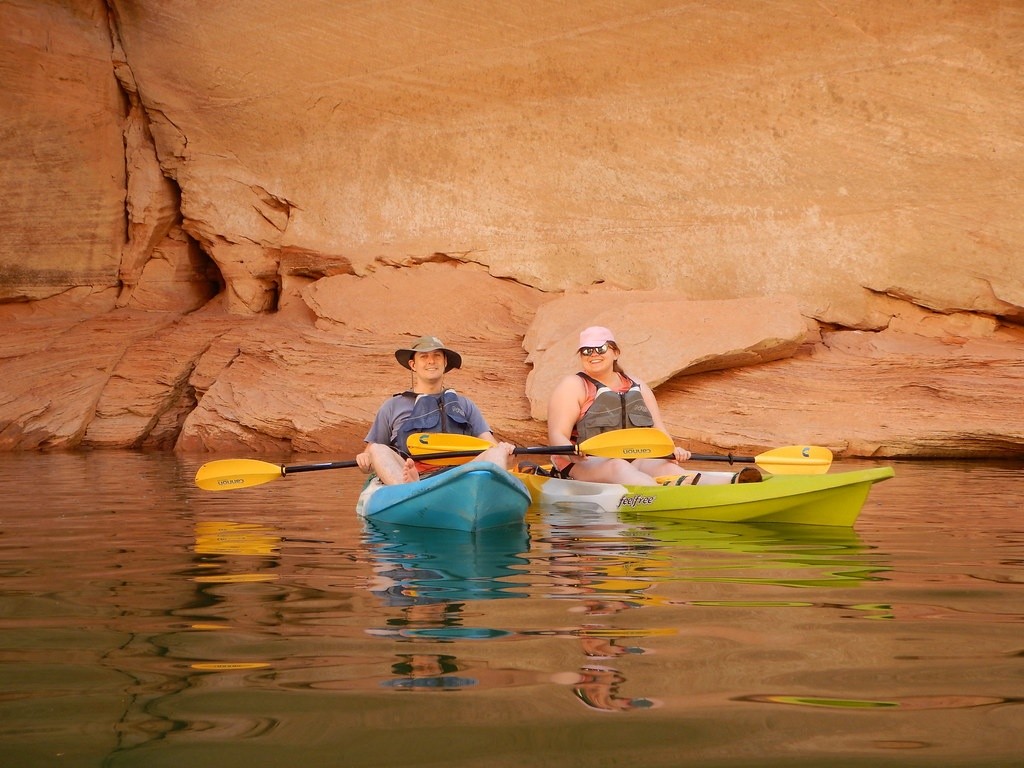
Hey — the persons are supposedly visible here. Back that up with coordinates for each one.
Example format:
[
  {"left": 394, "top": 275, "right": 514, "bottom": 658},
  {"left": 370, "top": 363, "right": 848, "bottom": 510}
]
[
  {"left": 546, "top": 326, "right": 763, "bottom": 487},
  {"left": 356, "top": 335, "right": 516, "bottom": 488}
]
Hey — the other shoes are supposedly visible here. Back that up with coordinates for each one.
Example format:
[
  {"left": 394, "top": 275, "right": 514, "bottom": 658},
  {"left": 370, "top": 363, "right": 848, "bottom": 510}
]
[{"left": 738, "top": 466, "right": 762, "bottom": 483}]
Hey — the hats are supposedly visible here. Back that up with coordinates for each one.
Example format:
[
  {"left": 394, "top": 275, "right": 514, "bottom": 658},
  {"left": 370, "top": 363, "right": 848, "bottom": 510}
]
[
  {"left": 576, "top": 326, "right": 616, "bottom": 354},
  {"left": 395, "top": 336, "right": 461, "bottom": 374}
]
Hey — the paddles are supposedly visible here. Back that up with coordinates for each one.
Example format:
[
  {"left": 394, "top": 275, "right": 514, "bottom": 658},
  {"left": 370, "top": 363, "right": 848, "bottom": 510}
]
[
  {"left": 195, "top": 426, "right": 678, "bottom": 493},
  {"left": 405, "top": 427, "right": 836, "bottom": 476}
]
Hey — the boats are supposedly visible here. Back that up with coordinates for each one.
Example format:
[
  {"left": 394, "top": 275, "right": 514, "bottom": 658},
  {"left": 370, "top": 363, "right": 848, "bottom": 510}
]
[
  {"left": 507, "top": 462, "right": 895, "bottom": 527},
  {"left": 354, "top": 458, "right": 533, "bottom": 532}
]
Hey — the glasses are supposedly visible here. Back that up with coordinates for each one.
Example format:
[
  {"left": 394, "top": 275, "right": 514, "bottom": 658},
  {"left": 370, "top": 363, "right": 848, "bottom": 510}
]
[{"left": 580, "top": 343, "right": 614, "bottom": 357}]
